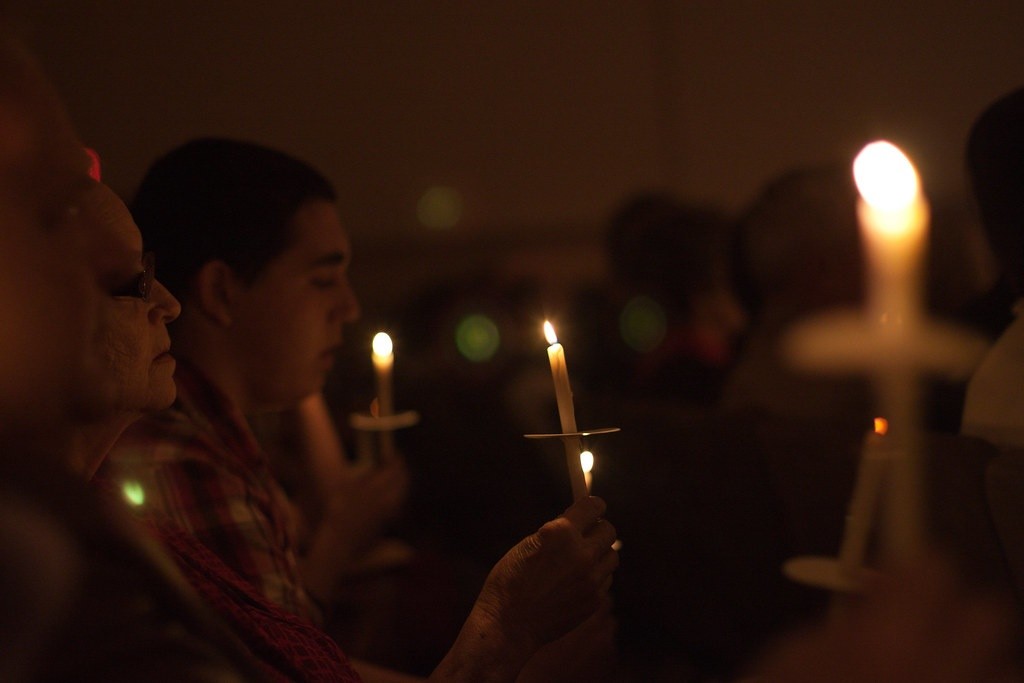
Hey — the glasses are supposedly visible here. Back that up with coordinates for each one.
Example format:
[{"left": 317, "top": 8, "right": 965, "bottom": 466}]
[{"left": 109, "top": 251, "right": 157, "bottom": 299}]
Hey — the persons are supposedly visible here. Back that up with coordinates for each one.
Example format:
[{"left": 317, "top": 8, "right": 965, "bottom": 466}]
[
  {"left": 0, "top": 87, "right": 1024, "bottom": 683},
  {"left": 0, "top": 169, "right": 619, "bottom": 683}
]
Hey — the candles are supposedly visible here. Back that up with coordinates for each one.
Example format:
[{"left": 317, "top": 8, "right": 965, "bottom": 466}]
[
  {"left": 541, "top": 318, "right": 588, "bottom": 503},
  {"left": 371, "top": 331, "right": 395, "bottom": 465},
  {"left": 840, "top": 416, "right": 889, "bottom": 565},
  {"left": 851, "top": 137, "right": 933, "bottom": 554}
]
